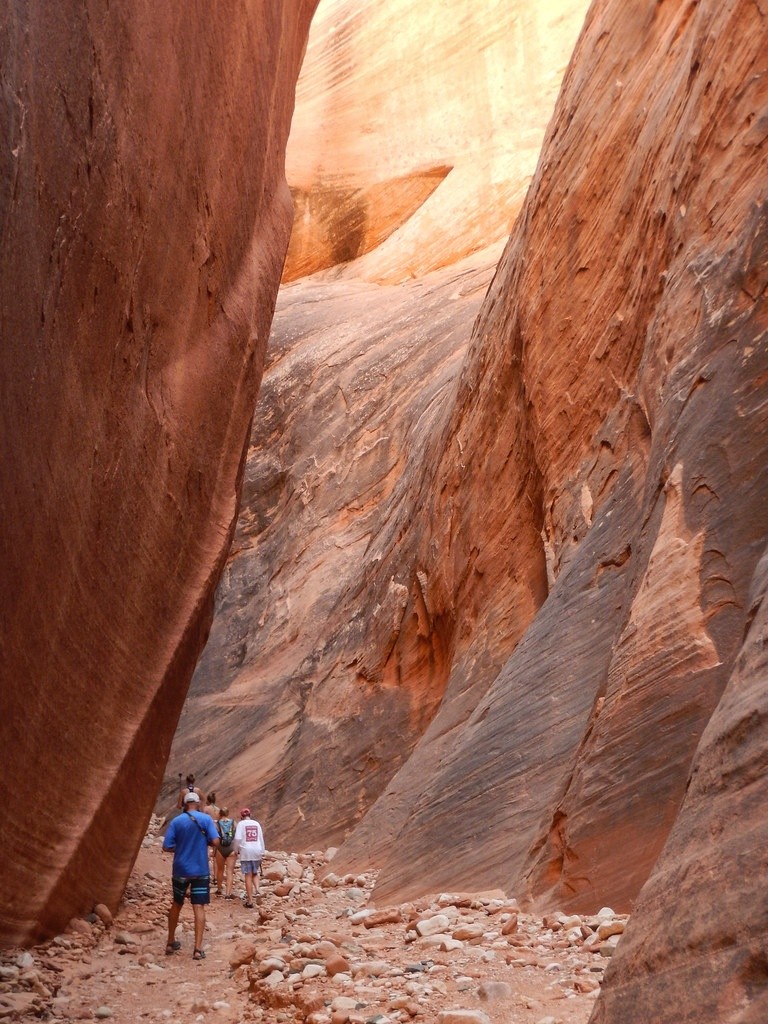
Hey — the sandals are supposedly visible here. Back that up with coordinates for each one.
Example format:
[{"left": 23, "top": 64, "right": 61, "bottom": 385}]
[
  {"left": 193, "top": 947, "right": 206, "bottom": 959},
  {"left": 166, "top": 941, "right": 180, "bottom": 955}
]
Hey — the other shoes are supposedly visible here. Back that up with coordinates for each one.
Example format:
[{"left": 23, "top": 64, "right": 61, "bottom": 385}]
[
  {"left": 211, "top": 879, "right": 218, "bottom": 884},
  {"left": 215, "top": 890, "right": 223, "bottom": 897},
  {"left": 225, "top": 896, "right": 234, "bottom": 900}
]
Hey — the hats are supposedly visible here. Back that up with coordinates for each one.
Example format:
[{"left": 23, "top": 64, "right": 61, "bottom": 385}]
[
  {"left": 184, "top": 793, "right": 200, "bottom": 803},
  {"left": 240, "top": 808, "right": 251, "bottom": 815}
]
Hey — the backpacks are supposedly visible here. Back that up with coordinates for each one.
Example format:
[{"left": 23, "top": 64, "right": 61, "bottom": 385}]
[{"left": 217, "top": 819, "right": 233, "bottom": 847}]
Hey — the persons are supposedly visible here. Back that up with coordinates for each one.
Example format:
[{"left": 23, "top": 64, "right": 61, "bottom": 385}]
[
  {"left": 213, "top": 807, "right": 240, "bottom": 900},
  {"left": 176, "top": 773, "right": 205, "bottom": 813},
  {"left": 202, "top": 793, "right": 221, "bottom": 886},
  {"left": 162, "top": 793, "right": 221, "bottom": 960},
  {"left": 233, "top": 808, "right": 265, "bottom": 908}
]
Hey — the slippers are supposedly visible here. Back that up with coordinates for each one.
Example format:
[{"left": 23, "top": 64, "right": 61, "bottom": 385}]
[
  {"left": 254, "top": 893, "right": 260, "bottom": 895},
  {"left": 243, "top": 902, "right": 254, "bottom": 908}
]
